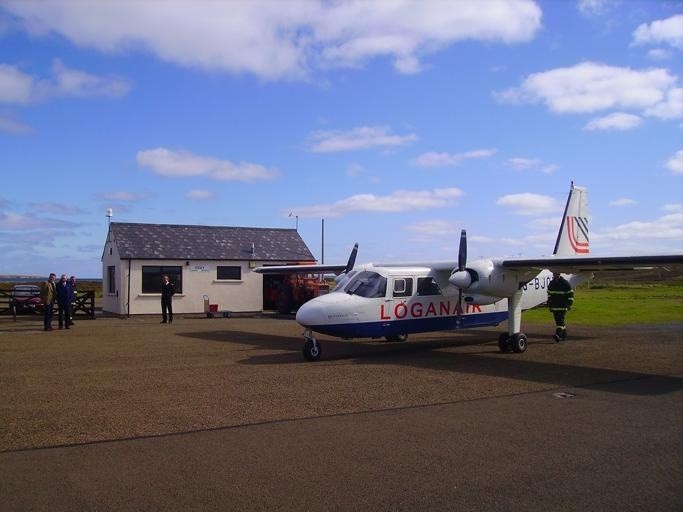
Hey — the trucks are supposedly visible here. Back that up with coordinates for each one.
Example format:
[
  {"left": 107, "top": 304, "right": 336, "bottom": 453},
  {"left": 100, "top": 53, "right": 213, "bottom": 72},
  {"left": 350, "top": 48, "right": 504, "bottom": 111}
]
[{"left": 263, "top": 262, "right": 329, "bottom": 314}]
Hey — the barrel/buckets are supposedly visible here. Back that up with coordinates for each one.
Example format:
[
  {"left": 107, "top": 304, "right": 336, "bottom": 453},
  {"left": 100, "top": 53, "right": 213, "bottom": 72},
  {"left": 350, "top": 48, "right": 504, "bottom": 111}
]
[{"left": 209, "top": 303, "right": 218, "bottom": 312}]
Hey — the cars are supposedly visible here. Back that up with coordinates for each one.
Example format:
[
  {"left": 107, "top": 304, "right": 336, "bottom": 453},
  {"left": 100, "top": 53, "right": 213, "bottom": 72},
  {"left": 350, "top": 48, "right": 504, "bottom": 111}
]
[{"left": 9, "top": 285, "right": 46, "bottom": 314}]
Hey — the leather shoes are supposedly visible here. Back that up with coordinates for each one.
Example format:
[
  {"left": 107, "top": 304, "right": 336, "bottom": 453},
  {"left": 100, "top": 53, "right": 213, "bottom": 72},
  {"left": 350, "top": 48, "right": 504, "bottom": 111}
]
[
  {"left": 159, "top": 319, "right": 174, "bottom": 324},
  {"left": 43, "top": 321, "right": 75, "bottom": 331}
]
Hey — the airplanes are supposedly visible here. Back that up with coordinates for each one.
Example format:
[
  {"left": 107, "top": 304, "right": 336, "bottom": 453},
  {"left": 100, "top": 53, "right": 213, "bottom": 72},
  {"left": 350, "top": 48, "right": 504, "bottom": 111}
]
[{"left": 253, "top": 180, "right": 683, "bottom": 364}]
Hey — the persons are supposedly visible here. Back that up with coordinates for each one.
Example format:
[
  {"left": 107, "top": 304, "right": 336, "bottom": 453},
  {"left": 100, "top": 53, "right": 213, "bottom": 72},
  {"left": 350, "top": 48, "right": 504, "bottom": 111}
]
[
  {"left": 38, "top": 273, "right": 79, "bottom": 331},
  {"left": 546, "top": 273, "right": 575, "bottom": 341},
  {"left": 160, "top": 275, "right": 175, "bottom": 325}
]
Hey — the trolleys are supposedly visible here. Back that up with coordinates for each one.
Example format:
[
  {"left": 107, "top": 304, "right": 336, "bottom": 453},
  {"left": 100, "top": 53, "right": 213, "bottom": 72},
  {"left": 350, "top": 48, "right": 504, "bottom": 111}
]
[{"left": 203, "top": 295, "right": 232, "bottom": 318}]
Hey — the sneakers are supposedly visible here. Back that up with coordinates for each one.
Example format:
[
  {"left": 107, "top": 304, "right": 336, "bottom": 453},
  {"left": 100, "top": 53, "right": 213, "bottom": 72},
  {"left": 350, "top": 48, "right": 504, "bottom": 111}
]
[{"left": 554, "top": 333, "right": 566, "bottom": 342}]
